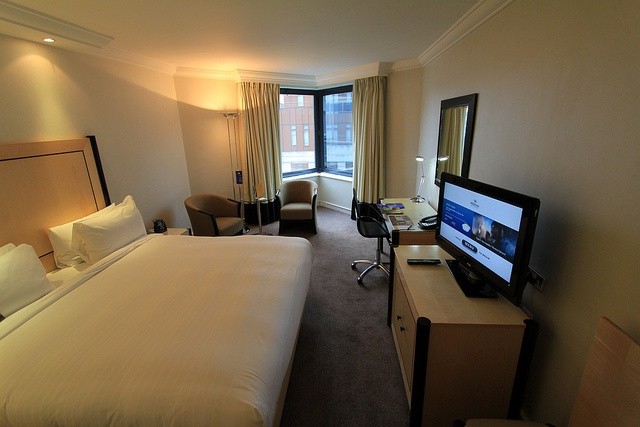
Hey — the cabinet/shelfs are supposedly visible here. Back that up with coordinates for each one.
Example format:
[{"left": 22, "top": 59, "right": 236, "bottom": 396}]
[{"left": 386, "top": 243, "right": 536, "bottom": 426}]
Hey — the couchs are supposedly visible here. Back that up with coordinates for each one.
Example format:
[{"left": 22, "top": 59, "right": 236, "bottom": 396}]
[
  {"left": 275, "top": 178, "right": 318, "bottom": 234},
  {"left": 183, "top": 192, "right": 244, "bottom": 235}
]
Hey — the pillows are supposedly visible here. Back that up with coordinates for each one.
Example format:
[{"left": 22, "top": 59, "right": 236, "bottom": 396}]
[
  {"left": 73, "top": 195, "right": 147, "bottom": 265},
  {"left": 46, "top": 201, "right": 116, "bottom": 267},
  {"left": 1, "top": 242, "right": 16, "bottom": 254},
  {"left": 1, "top": 243, "right": 56, "bottom": 318}
]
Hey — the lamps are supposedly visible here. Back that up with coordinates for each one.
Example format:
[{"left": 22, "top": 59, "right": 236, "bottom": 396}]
[
  {"left": 437, "top": 155, "right": 451, "bottom": 161},
  {"left": 409, "top": 155, "right": 427, "bottom": 202},
  {"left": 219, "top": 110, "right": 250, "bottom": 234}
]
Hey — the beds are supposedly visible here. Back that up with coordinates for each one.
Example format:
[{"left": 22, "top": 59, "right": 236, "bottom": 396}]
[{"left": 1, "top": 134, "right": 314, "bottom": 425}]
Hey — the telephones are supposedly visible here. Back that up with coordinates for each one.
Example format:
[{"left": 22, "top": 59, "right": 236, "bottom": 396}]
[{"left": 417, "top": 214, "right": 437, "bottom": 230}]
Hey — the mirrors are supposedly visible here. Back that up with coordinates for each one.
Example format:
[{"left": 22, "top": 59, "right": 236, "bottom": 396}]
[{"left": 434, "top": 92, "right": 479, "bottom": 187}]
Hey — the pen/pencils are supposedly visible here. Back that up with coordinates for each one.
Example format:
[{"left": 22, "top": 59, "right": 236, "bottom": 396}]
[{"left": 407, "top": 225, "right": 412, "bottom": 230}]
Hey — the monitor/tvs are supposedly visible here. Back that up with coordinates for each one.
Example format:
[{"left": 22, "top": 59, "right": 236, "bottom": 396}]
[{"left": 434, "top": 171, "right": 541, "bottom": 308}]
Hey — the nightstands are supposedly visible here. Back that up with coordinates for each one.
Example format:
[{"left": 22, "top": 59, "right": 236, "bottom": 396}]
[{"left": 146, "top": 227, "right": 189, "bottom": 236}]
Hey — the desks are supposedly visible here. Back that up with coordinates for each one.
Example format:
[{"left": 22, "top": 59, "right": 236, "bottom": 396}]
[
  {"left": 379, "top": 196, "right": 438, "bottom": 252},
  {"left": 229, "top": 189, "right": 279, "bottom": 235}
]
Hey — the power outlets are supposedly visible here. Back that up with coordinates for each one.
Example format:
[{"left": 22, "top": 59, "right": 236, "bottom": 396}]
[{"left": 528, "top": 265, "right": 545, "bottom": 293}]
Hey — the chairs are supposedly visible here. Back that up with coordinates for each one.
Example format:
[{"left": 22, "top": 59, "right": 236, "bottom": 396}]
[
  {"left": 350, "top": 187, "right": 390, "bottom": 284},
  {"left": 465, "top": 315, "right": 640, "bottom": 427}
]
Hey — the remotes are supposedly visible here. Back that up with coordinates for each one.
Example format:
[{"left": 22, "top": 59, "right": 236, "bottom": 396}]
[{"left": 407, "top": 257, "right": 441, "bottom": 266}]
